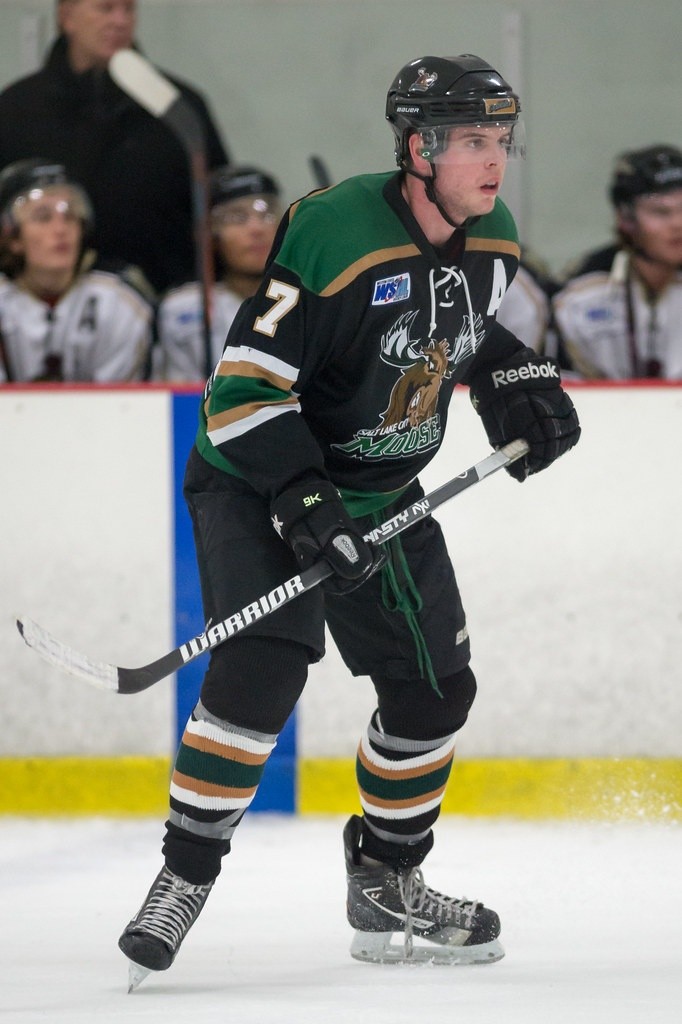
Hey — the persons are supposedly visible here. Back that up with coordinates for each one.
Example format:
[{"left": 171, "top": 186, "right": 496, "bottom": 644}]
[
  {"left": 150, "top": 166, "right": 286, "bottom": 381},
  {"left": 0, "top": 157, "right": 154, "bottom": 386},
  {"left": 551, "top": 143, "right": 682, "bottom": 379},
  {"left": 117, "top": 52, "right": 581, "bottom": 991},
  {"left": 0, "top": 0, "right": 230, "bottom": 345}
]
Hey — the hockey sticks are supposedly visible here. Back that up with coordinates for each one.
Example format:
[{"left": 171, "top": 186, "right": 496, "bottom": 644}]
[{"left": 9, "top": 434, "right": 530, "bottom": 699}]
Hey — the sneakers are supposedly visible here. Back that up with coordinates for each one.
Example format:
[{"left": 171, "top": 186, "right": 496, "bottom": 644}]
[
  {"left": 118, "top": 864, "right": 217, "bottom": 994},
  {"left": 344, "top": 815, "right": 505, "bottom": 964}
]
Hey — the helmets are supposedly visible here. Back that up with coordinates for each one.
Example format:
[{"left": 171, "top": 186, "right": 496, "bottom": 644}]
[
  {"left": 208, "top": 164, "right": 279, "bottom": 208},
  {"left": 0, "top": 159, "right": 83, "bottom": 211},
  {"left": 609, "top": 145, "right": 682, "bottom": 207},
  {"left": 386, "top": 55, "right": 521, "bottom": 166}
]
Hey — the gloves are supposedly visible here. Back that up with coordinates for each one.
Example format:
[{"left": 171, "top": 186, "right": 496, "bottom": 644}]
[
  {"left": 459, "top": 321, "right": 581, "bottom": 482},
  {"left": 270, "top": 479, "right": 389, "bottom": 595}
]
